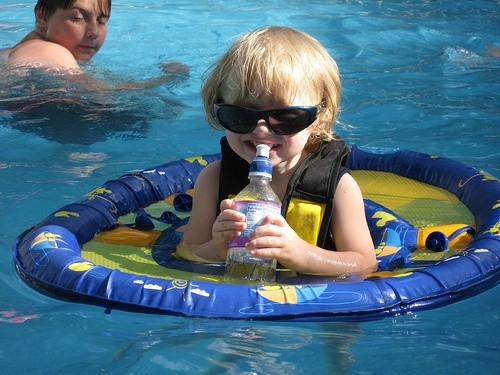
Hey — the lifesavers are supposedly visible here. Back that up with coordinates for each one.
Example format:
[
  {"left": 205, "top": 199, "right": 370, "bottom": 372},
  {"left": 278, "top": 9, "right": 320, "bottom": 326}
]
[{"left": 12, "top": 144, "right": 500, "bottom": 321}]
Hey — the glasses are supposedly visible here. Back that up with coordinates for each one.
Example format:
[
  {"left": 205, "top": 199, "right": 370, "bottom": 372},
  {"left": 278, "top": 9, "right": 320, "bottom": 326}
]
[{"left": 213, "top": 100, "right": 326, "bottom": 136}]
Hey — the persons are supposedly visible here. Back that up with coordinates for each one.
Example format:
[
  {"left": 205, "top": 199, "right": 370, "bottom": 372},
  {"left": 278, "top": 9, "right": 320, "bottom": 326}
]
[
  {"left": 0, "top": 0, "right": 189, "bottom": 96},
  {"left": 183, "top": 25, "right": 380, "bottom": 280}
]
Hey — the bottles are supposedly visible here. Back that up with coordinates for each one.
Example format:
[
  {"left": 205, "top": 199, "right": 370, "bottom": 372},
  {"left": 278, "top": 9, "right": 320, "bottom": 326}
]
[{"left": 222, "top": 145, "right": 281, "bottom": 290}]
